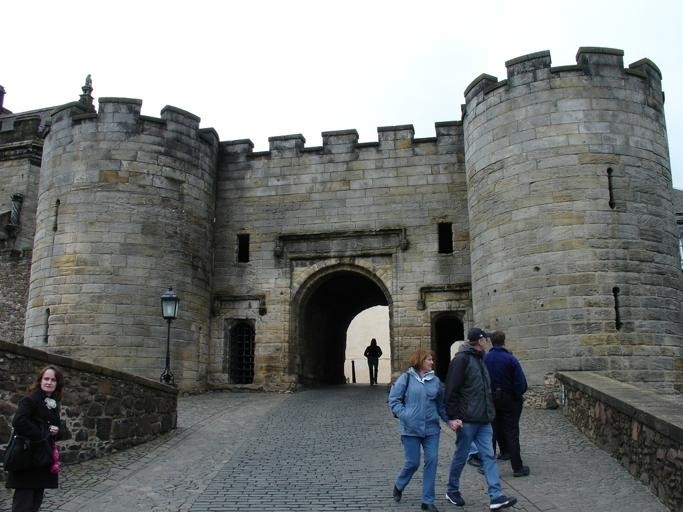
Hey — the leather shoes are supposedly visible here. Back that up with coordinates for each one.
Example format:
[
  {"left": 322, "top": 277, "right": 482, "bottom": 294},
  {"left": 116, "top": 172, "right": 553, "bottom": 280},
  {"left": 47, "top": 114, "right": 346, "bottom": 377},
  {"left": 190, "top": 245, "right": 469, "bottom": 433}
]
[
  {"left": 420, "top": 502, "right": 439, "bottom": 512},
  {"left": 391, "top": 483, "right": 402, "bottom": 502}
]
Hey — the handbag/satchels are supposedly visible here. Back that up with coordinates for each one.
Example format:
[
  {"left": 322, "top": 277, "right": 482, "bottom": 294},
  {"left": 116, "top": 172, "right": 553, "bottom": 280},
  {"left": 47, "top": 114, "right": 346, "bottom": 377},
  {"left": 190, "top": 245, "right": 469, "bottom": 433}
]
[
  {"left": 386, "top": 382, "right": 405, "bottom": 418},
  {"left": 2, "top": 432, "right": 55, "bottom": 474}
]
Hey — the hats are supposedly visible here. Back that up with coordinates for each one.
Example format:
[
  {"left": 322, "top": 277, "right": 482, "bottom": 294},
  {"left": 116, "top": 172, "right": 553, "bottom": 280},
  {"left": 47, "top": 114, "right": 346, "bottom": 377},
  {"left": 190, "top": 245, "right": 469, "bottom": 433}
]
[{"left": 467, "top": 326, "right": 493, "bottom": 342}]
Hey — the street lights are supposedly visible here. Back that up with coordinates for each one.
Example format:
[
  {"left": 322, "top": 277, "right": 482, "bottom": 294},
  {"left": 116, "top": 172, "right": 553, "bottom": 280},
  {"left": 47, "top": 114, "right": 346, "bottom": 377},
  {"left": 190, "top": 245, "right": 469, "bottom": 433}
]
[{"left": 159, "top": 286, "right": 179, "bottom": 386}]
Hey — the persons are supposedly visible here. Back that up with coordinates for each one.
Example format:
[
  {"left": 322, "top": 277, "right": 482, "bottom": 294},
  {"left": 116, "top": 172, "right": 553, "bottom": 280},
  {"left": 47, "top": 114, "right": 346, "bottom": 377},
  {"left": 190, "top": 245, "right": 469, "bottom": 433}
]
[
  {"left": 362, "top": 339, "right": 383, "bottom": 387},
  {"left": 1, "top": 364, "right": 73, "bottom": 511},
  {"left": 445, "top": 327, "right": 517, "bottom": 512},
  {"left": 388, "top": 347, "right": 462, "bottom": 511},
  {"left": 484, "top": 330, "right": 530, "bottom": 478},
  {"left": 467, "top": 334, "right": 496, "bottom": 474}
]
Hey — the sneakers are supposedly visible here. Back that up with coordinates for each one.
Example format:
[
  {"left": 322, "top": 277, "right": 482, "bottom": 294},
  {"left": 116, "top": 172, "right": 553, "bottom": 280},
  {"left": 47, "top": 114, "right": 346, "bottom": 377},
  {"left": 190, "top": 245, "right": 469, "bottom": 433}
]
[
  {"left": 487, "top": 494, "right": 518, "bottom": 510},
  {"left": 511, "top": 465, "right": 530, "bottom": 477},
  {"left": 444, "top": 490, "right": 465, "bottom": 506},
  {"left": 479, "top": 465, "right": 485, "bottom": 474}
]
